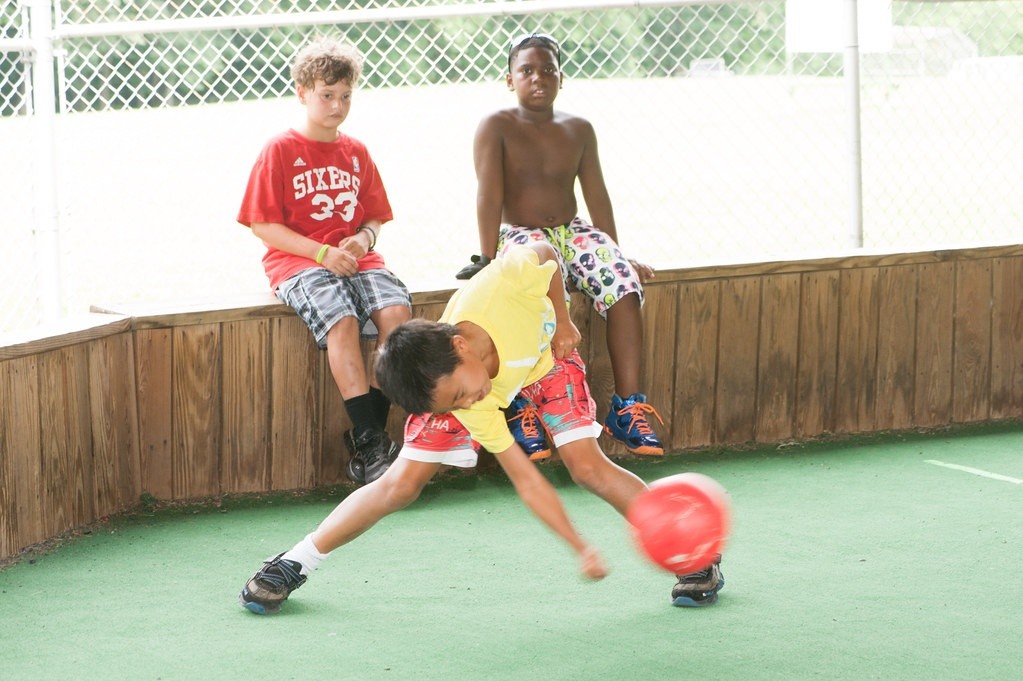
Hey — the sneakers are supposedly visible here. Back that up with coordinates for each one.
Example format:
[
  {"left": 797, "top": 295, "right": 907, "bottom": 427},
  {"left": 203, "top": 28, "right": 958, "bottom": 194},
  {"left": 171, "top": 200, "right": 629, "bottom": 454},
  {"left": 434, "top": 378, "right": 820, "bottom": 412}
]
[
  {"left": 511, "top": 399, "right": 552, "bottom": 465},
  {"left": 671, "top": 553, "right": 724, "bottom": 606},
  {"left": 243, "top": 549, "right": 308, "bottom": 617},
  {"left": 358, "top": 426, "right": 400, "bottom": 484},
  {"left": 600, "top": 392, "right": 665, "bottom": 457},
  {"left": 342, "top": 426, "right": 364, "bottom": 482}
]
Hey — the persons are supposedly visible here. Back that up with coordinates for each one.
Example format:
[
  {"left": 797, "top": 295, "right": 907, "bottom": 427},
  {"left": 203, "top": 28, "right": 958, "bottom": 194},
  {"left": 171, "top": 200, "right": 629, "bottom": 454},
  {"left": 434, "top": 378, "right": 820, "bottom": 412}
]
[
  {"left": 236, "top": 35, "right": 412, "bottom": 484},
  {"left": 457, "top": 32, "right": 666, "bottom": 458},
  {"left": 238, "top": 240, "right": 723, "bottom": 617}
]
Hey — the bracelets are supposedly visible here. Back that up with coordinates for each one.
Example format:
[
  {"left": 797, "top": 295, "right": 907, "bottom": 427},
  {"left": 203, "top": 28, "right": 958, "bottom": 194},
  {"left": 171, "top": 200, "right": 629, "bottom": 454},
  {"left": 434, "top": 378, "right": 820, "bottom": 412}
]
[
  {"left": 357, "top": 226, "right": 376, "bottom": 250},
  {"left": 317, "top": 244, "right": 329, "bottom": 264}
]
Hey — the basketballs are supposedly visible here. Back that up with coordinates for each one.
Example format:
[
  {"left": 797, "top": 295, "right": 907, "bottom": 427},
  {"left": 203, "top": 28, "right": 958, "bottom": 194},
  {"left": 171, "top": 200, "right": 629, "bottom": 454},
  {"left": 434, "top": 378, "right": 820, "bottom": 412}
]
[{"left": 630, "top": 471, "right": 724, "bottom": 573}]
